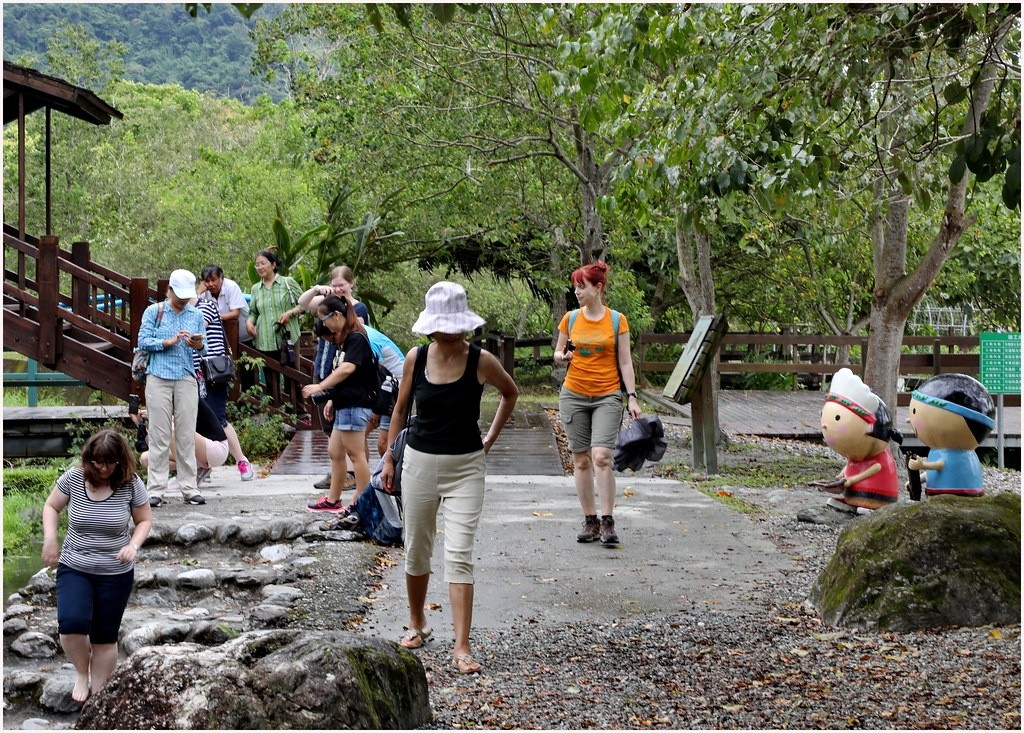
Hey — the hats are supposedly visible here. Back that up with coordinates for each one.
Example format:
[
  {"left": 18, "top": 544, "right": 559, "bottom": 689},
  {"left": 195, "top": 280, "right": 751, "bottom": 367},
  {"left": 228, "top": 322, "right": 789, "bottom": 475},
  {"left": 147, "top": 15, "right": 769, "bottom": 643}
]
[
  {"left": 411, "top": 281, "right": 486, "bottom": 335},
  {"left": 169, "top": 269, "right": 196, "bottom": 299}
]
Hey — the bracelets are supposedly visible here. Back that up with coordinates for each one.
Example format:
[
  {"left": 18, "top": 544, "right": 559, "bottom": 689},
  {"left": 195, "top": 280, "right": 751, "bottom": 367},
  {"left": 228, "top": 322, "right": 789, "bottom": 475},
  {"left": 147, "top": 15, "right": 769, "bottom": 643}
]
[
  {"left": 133, "top": 544, "right": 138, "bottom": 550},
  {"left": 289, "top": 310, "right": 295, "bottom": 317},
  {"left": 314, "top": 285, "right": 320, "bottom": 292}
]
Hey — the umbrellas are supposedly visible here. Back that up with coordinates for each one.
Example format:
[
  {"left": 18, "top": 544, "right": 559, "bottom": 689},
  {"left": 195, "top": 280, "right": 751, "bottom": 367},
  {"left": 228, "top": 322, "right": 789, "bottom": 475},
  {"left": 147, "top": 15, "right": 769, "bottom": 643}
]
[{"left": 611, "top": 405, "right": 667, "bottom": 472}]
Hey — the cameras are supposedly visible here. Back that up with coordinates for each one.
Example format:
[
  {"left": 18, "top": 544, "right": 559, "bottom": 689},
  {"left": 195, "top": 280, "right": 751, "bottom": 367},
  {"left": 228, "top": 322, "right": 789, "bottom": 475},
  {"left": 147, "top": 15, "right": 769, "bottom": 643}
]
[{"left": 564, "top": 338, "right": 577, "bottom": 354}]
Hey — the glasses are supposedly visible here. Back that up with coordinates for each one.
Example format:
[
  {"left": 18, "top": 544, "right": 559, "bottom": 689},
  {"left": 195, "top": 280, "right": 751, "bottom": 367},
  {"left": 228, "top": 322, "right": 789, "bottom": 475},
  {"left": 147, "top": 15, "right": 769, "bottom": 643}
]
[
  {"left": 254, "top": 262, "right": 270, "bottom": 269},
  {"left": 330, "top": 334, "right": 335, "bottom": 347},
  {"left": 320, "top": 312, "right": 342, "bottom": 323},
  {"left": 91, "top": 460, "right": 119, "bottom": 469}
]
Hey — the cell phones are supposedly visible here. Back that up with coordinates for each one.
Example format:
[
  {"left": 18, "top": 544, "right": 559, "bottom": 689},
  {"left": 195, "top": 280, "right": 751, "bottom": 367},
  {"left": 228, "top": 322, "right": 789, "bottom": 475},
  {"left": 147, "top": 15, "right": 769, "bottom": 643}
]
[{"left": 191, "top": 333, "right": 203, "bottom": 339}]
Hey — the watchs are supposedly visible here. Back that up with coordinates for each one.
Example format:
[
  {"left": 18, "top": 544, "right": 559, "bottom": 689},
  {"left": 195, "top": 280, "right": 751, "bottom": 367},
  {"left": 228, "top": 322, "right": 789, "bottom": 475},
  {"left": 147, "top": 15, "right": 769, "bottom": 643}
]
[{"left": 627, "top": 392, "right": 637, "bottom": 398}]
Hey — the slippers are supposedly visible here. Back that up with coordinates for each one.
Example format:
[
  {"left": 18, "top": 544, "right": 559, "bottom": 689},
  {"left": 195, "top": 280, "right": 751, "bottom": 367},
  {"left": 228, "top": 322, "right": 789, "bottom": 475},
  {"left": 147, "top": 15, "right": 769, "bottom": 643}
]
[
  {"left": 400, "top": 628, "right": 431, "bottom": 648},
  {"left": 452, "top": 652, "right": 481, "bottom": 675}
]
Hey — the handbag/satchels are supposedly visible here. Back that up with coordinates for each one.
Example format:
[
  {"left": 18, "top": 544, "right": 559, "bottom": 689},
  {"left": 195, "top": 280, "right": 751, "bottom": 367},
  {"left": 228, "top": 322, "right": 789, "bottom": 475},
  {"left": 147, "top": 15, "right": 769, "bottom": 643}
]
[
  {"left": 202, "top": 355, "right": 233, "bottom": 383},
  {"left": 132, "top": 302, "right": 164, "bottom": 384},
  {"left": 357, "top": 481, "right": 403, "bottom": 547},
  {"left": 371, "top": 415, "right": 418, "bottom": 496}
]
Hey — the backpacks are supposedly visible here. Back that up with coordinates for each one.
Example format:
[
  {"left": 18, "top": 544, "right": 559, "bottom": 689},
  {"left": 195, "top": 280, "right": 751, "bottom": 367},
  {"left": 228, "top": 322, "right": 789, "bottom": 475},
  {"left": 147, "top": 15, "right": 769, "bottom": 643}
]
[{"left": 351, "top": 331, "right": 399, "bottom": 417}]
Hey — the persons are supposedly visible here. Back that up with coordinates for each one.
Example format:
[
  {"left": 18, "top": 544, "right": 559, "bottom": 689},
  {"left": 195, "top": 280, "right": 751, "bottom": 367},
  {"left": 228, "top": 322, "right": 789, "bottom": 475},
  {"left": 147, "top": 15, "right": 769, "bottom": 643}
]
[
  {"left": 187, "top": 297, "right": 254, "bottom": 481},
  {"left": 309, "top": 318, "right": 406, "bottom": 462},
  {"left": 554, "top": 260, "right": 641, "bottom": 545},
  {"left": 297, "top": 266, "right": 368, "bottom": 491},
  {"left": 128, "top": 368, "right": 229, "bottom": 488},
  {"left": 818, "top": 367, "right": 903, "bottom": 513},
  {"left": 137, "top": 268, "right": 208, "bottom": 507},
  {"left": 379, "top": 281, "right": 519, "bottom": 673},
  {"left": 42, "top": 429, "right": 152, "bottom": 701},
  {"left": 197, "top": 264, "right": 261, "bottom": 401},
  {"left": 245, "top": 251, "right": 312, "bottom": 419},
  {"left": 302, "top": 295, "right": 379, "bottom": 512},
  {"left": 905, "top": 373, "right": 996, "bottom": 496}
]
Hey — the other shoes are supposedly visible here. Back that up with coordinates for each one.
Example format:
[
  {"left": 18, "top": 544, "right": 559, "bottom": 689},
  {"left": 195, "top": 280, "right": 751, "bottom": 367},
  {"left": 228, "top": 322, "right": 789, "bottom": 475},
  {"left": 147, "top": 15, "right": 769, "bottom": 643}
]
[{"left": 197, "top": 467, "right": 212, "bottom": 486}]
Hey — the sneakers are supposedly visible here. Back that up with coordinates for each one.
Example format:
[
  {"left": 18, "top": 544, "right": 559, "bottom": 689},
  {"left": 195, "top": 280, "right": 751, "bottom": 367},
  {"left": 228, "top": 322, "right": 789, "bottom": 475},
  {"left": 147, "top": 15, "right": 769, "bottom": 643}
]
[
  {"left": 307, "top": 497, "right": 344, "bottom": 513},
  {"left": 183, "top": 495, "right": 205, "bottom": 505},
  {"left": 237, "top": 460, "right": 254, "bottom": 480},
  {"left": 314, "top": 471, "right": 331, "bottom": 489},
  {"left": 576, "top": 519, "right": 600, "bottom": 541},
  {"left": 149, "top": 496, "right": 162, "bottom": 507},
  {"left": 600, "top": 517, "right": 619, "bottom": 543},
  {"left": 342, "top": 473, "right": 356, "bottom": 491}
]
